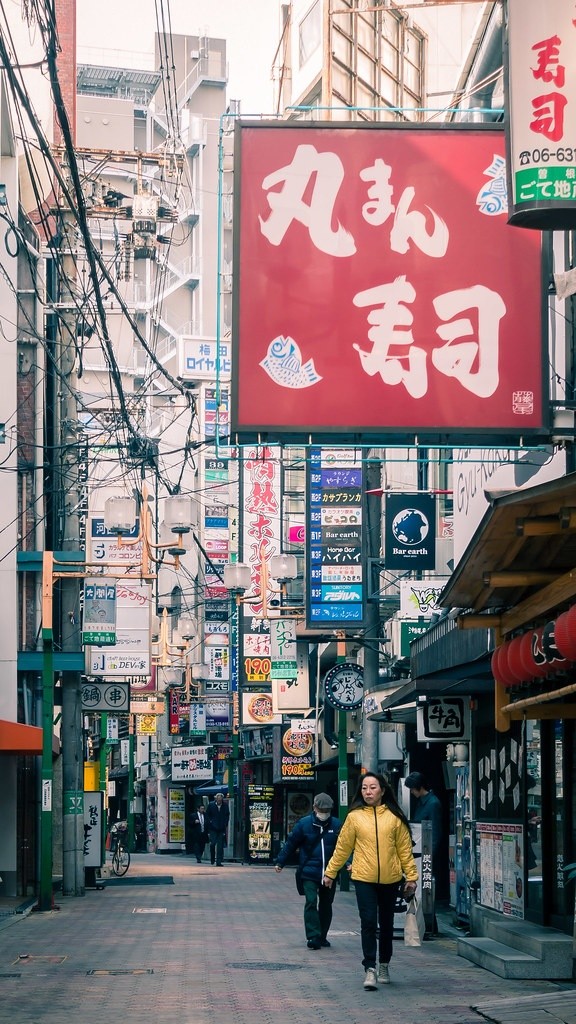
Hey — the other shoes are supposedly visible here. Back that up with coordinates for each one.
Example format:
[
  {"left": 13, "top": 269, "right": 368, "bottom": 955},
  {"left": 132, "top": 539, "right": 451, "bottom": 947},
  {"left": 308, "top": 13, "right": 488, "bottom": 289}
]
[
  {"left": 320, "top": 938, "right": 330, "bottom": 947},
  {"left": 307, "top": 938, "right": 321, "bottom": 950},
  {"left": 211, "top": 860, "right": 215, "bottom": 865},
  {"left": 197, "top": 859, "right": 201, "bottom": 863},
  {"left": 216, "top": 863, "right": 224, "bottom": 866}
]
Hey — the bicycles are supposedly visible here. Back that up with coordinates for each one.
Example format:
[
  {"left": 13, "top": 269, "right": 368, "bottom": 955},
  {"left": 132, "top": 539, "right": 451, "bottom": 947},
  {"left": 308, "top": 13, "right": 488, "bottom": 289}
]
[{"left": 109, "top": 824, "right": 131, "bottom": 877}]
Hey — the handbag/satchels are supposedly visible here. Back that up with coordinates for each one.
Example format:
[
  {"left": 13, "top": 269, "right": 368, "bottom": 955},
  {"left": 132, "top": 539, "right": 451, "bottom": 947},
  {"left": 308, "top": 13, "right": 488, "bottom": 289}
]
[
  {"left": 295, "top": 868, "right": 306, "bottom": 895},
  {"left": 404, "top": 892, "right": 426, "bottom": 947}
]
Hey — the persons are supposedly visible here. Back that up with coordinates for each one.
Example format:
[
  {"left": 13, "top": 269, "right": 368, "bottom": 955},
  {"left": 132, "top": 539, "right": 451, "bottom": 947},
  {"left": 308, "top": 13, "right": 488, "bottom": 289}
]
[
  {"left": 190, "top": 805, "right": 208, "bottom": 863},
  {"left": 324, "top": 772, "right": 419, "bottom": 991},
  {"left": 275, "top": 793, "right": 352, "bottom": 949},
  {"left": 206, "top": 793, "right": 230, "bottom": 865},
  {"left": 405, "top": 772, "right": 441, "bottom": 936}
]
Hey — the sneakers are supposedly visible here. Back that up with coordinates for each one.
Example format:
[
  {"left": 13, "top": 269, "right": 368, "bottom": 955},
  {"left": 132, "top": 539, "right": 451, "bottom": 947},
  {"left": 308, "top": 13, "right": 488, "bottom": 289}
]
[
  {"left": 377, "top": 963, "right": 390, "bottom": 984},
  {"left": 363, "top": 968, "right": 378, "bottom": 989}
]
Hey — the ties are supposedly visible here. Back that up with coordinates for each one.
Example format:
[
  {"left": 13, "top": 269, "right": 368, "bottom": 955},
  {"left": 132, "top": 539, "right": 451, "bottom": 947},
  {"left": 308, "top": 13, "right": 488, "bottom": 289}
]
[{"left": 200, "top": 813, "right": 203, "bottom": 823}]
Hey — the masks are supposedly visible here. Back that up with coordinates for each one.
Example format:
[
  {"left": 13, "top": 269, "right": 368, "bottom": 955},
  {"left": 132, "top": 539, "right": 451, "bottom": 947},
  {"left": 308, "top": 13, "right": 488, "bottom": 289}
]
[{"left": 315, "top": 810, "right": 330, "bottom": 821}]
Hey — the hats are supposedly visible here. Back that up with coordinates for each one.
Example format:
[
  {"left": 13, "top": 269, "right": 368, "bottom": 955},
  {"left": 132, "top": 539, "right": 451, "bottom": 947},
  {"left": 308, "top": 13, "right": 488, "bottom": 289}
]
[{"left": 314, "top": 792, "right": 333, "bottom": 808}]
